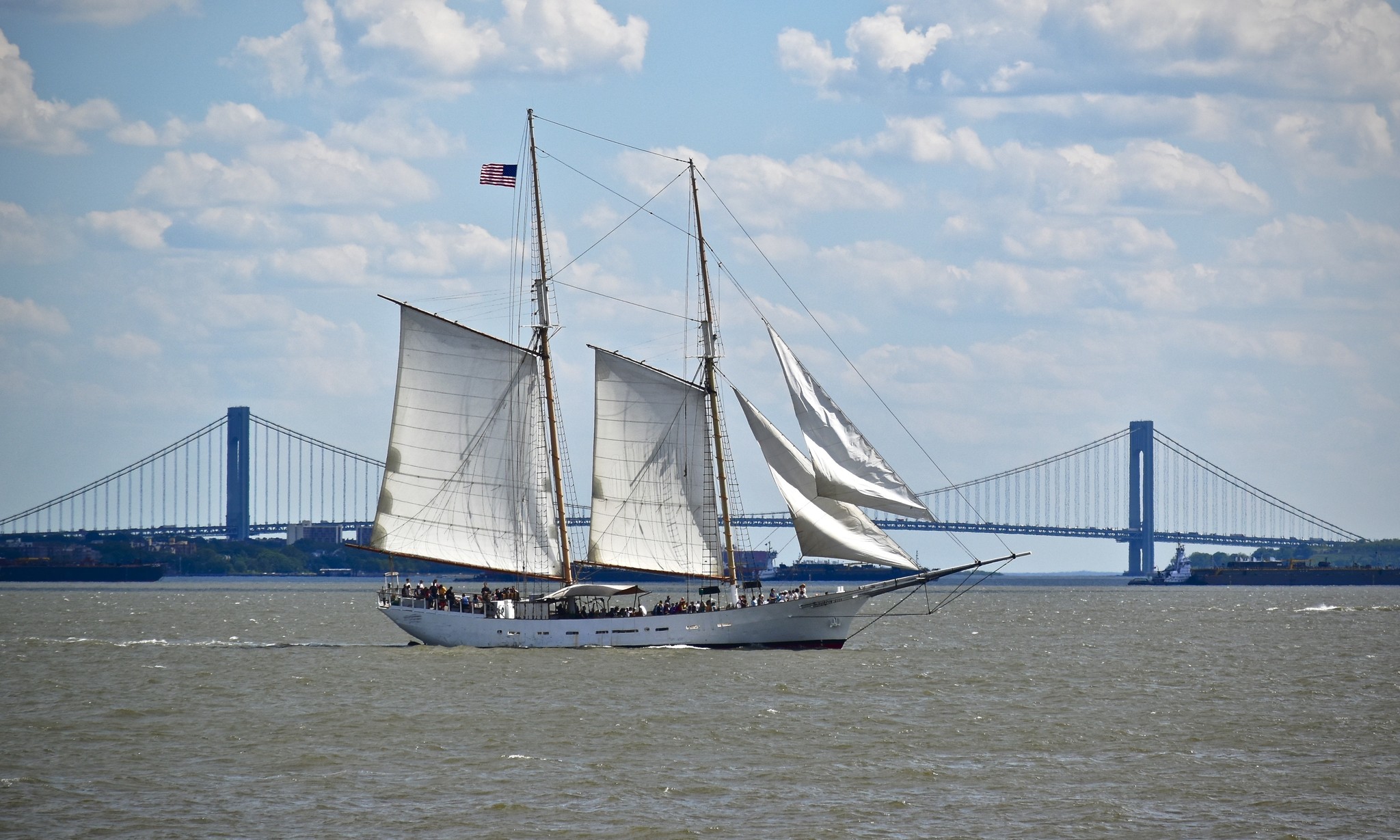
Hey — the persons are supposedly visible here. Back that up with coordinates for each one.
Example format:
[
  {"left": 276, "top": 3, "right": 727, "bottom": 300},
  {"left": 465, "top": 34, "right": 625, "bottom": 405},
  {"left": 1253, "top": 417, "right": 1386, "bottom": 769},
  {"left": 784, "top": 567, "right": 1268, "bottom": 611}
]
[
  {"left": 558, "top": 604, "right": 563, "bottom": 618},
  {"left": 481, "top": 583, "right": 518, "bottom": 608},
  {"left": 458, "top": 594, "right": 469, "bottom": 612},
  {"left": 609, "top": 607, "right": 635, "bottom": 617},
  {"left": 751, "top": 584, "right": 808, "bottom": 606},
  {"left": 401, "top": 579, "right": 412, "bottom": 598},
  {"left": 576, "top": 603, "right": 606, "bottom": 618},
  {"left": 473, "top": 594, "right": 479, "bottom": 603},
  {"left": 736, "top": 595, "right": 748, "bottom": 608},
  {"left": 638, "top": 604, "right": 647, "bottom": 616},
  {"left": 414, "top": 580, "right": 456, "bottom": 610},
  {"left": 652, "top": 596, "right": 716, "bottom": 615}
]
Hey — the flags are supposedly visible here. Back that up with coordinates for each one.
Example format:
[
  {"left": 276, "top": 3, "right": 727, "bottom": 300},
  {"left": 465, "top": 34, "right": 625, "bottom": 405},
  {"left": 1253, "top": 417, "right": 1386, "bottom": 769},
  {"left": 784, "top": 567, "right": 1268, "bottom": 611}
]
[{"left": 480, "top": 163, "right": 517, "bottom": 188}]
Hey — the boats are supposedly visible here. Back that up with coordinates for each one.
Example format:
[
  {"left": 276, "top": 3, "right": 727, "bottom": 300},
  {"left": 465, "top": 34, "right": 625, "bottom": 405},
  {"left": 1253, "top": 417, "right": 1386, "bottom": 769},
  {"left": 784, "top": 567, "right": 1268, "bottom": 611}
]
[{"left": 1127, "top": 540, "right": 1194, "bottom": 585}]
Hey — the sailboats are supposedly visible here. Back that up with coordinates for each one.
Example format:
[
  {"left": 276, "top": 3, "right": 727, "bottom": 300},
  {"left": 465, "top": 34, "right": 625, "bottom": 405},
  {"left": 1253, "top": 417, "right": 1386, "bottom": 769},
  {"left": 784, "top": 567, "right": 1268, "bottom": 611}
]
[{"left": 345, "top": 103, "right": 1030, "bottom": 648}]
